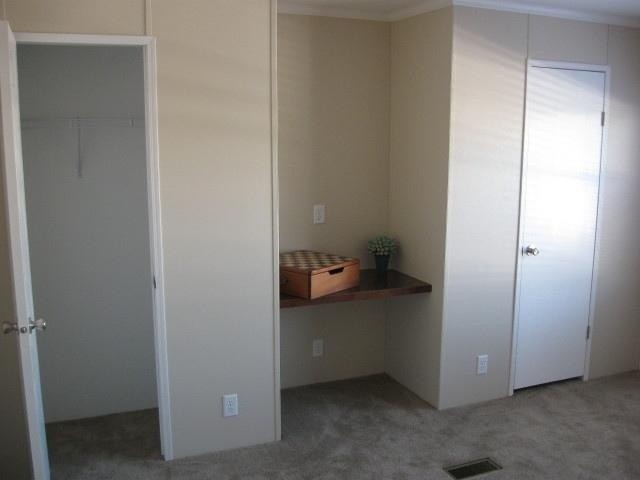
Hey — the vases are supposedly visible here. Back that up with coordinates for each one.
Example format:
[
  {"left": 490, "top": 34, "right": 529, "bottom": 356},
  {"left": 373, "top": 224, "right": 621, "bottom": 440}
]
[{"left": 376, "top": 255, "right": 390, "bottom": 273}]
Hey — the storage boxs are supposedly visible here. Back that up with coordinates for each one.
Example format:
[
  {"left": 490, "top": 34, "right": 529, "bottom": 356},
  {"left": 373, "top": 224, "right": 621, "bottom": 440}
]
[{"left": 279, "top": 250, "right": 361, "bottom": 300}]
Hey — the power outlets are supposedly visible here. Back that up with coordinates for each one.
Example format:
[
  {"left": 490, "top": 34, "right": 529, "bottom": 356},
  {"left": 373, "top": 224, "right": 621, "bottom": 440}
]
[{"left": 222, "top": 394, "right": 238, "bottom": 417}]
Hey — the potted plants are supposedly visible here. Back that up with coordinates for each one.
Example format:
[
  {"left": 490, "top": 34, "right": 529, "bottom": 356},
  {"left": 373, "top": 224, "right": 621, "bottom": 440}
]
[{"left": 366, "top": 235, "right": 400, "bottom": 256}]
